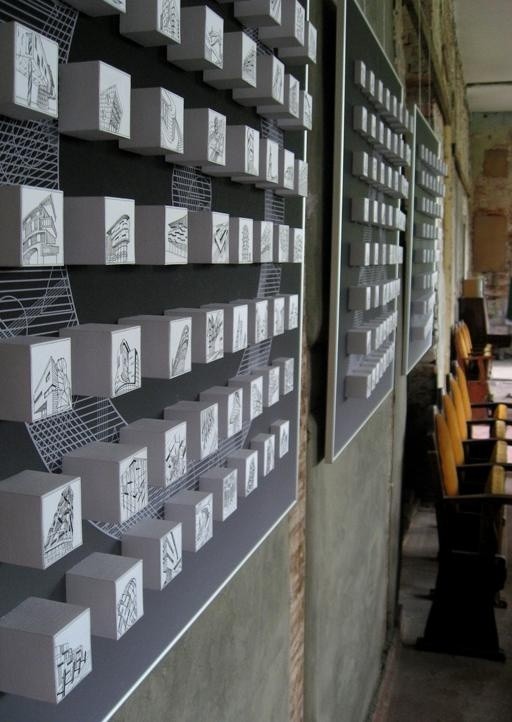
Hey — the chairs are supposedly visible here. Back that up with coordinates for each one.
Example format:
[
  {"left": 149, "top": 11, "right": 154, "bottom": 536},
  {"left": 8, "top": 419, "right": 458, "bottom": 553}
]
[{"left": 404, "top": 298, "right": 511, "bottom": 664}]
